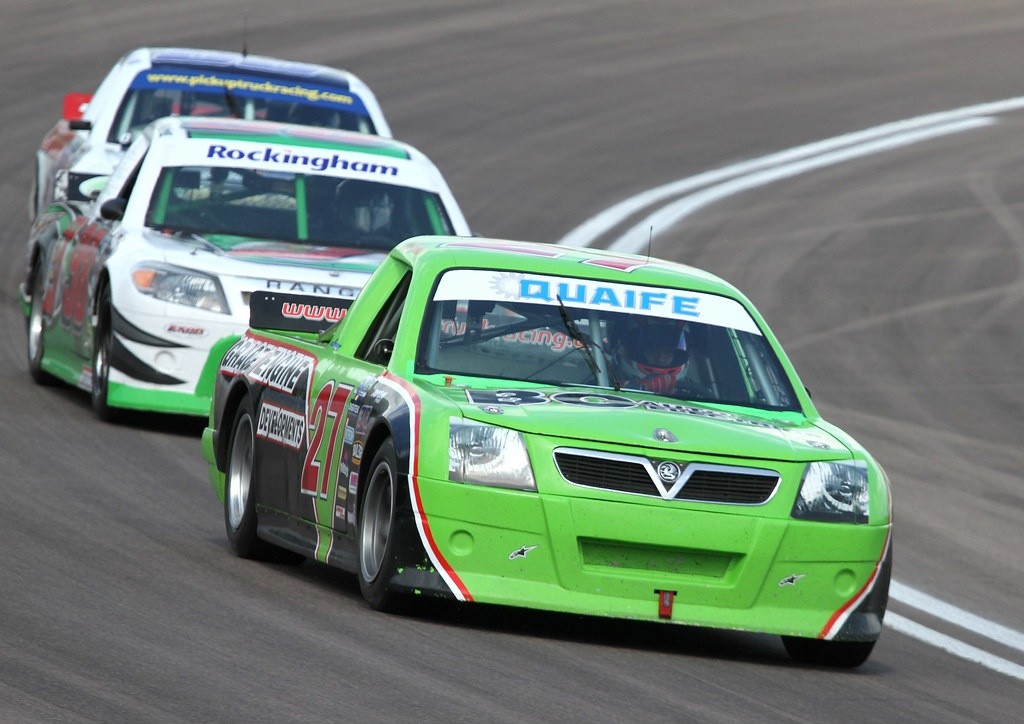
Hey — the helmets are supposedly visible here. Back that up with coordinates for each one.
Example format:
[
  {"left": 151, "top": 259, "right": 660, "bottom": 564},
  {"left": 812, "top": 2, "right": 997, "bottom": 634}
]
[
  {"left": 334, "top": 179, "right": 393, "bottom": 234},
  {"left": 606, "top": 312, "right": 692, "bottom": 396}
]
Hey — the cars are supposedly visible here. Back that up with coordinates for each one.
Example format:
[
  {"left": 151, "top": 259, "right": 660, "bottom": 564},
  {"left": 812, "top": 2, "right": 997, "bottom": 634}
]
[{"left": 31, "top": 46, "right": 395, "bottom": 221}]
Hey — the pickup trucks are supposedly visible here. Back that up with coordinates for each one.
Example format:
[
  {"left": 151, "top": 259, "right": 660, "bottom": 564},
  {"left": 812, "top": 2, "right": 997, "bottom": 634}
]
[
  {"left": 27, "top": 115, "right": 474, "bottom": 431},
  {"left": 198, "top": 235, "right": 896, "bottom": 669}
]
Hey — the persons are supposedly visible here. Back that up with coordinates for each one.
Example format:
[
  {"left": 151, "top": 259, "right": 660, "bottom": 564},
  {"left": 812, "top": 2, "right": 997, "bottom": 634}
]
[
  {"left": 312, "top": 178, "right": 414, "bottom": 252},
  {"left": 582, "top": 313, "right": 717, "bottom": 403}
]
